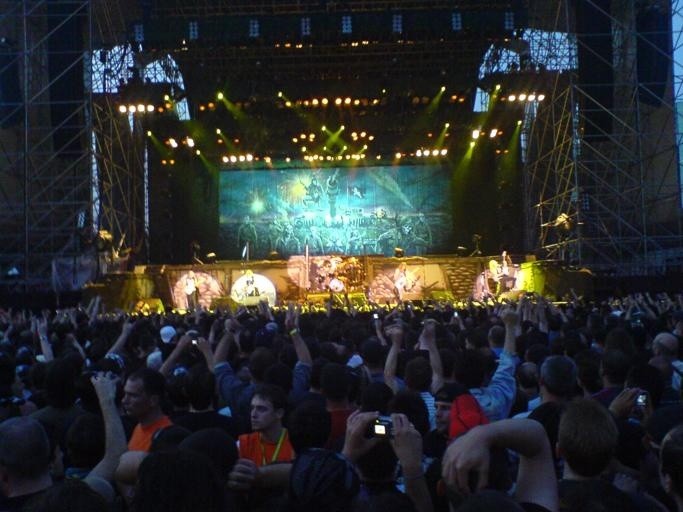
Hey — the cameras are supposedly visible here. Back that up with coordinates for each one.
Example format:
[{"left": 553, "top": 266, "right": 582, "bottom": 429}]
[
  {"left": 190, "top": 339, "right": 198, "bottom": 346},
  {"left": 633, "top": 391, "right": 648, "bottom": 409},
  {"left": 659, "top": 299, "right": 666, "bottom": 304},
  {"left": 366, "top": 416, "right": 396, "bottom": 443}
]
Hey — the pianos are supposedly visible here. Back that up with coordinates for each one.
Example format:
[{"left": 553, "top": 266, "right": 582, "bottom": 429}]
[{"left": 489, "top": 274, "right": 514, "bottom": 283}]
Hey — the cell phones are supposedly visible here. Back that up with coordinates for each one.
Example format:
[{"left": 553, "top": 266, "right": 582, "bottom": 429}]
[
  {"left": 454, "top": 311, "right": 458, "bottom": 319},
  {"left": 373, "top": 313, "right": 379, "bottom": 322}
]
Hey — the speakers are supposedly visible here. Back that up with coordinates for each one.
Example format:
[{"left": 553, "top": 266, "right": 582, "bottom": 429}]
[
  {"left": 632, "top": 0, "right": 671, "bottom": 109},
  {"left": 49, "top": 62, "right": 88, "bottom": 161},
  {"left": 0, "top": 35, "right": 26, "bottom": 129},
  {"left": 575, "top": 0, "right": 614, "bottom": 142}
]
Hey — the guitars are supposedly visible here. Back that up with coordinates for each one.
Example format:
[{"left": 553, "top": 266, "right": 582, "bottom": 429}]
[
  {"left": 183, "top": 278, "right": 206, "bottom": 294},
  {"left": 394, "top": 267, "right": 419, "bottom": 288}
]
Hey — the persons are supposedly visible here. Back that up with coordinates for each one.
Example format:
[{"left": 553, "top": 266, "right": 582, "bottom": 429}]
[
  {"left": 0, "top": 250, "right": 683, "bottom": 511},
  {"left": 231, "top": 212, "right": 434, "bottom": 260}
]
